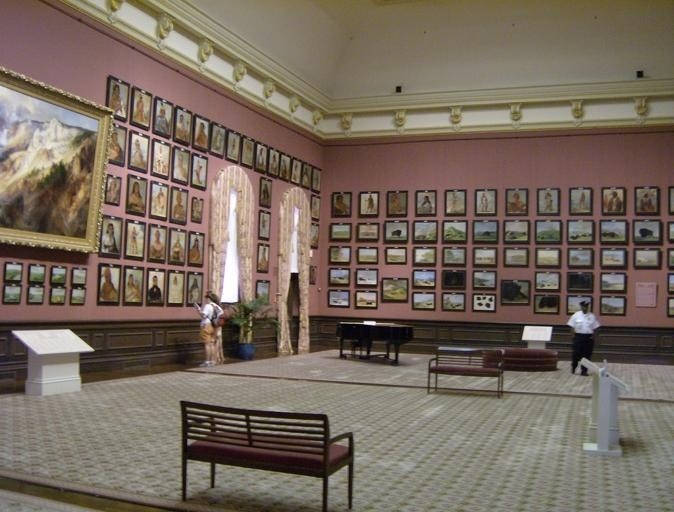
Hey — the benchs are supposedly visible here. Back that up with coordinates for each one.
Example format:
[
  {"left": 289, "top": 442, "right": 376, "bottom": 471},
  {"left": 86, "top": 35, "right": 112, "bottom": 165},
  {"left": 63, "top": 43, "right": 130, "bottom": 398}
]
[
  {"left": 479, "top": 344, "right": 558, "bottom": 373},
  {"left": 173, "top": 398, "right": 359, "bottom": 508},
  {"left": 422, "top": 343, "right": 508, "bottom": 399}
]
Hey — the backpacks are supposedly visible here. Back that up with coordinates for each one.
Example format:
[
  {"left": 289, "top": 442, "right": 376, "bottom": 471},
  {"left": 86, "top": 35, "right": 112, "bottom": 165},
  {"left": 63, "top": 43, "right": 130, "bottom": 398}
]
[{"left": 207, "top": 304, "right": 225, "bottom": 327}]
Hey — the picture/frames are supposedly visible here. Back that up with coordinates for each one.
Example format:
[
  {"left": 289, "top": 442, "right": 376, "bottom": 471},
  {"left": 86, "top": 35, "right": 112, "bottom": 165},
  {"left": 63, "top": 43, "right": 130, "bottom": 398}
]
[
  {"left": 255, "top": 194, "right": 272, "bottom": 300},
  {"left": 327, "top": 186, "right": 674, "bottom": 318},
  {"left": 94, "top": 75, "right": 212, "bottom": 308},
  {"left": 212, "top": 121, "right": 322, "bottom": 195},
  {"left": 3, "top": 261, "right": 92, "bottom": 307},
  {"left": 309, "top": 195, "right": 321, "bottom": 285},
  {"left": 0, "top": 64, "right": 118, "bottom": 254}
]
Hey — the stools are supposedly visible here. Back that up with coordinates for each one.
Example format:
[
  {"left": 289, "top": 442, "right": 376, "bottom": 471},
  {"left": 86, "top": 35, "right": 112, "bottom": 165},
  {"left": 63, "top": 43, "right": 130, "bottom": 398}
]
[{"left": 348, "top": 340, "right": 373, "bottom": 359}]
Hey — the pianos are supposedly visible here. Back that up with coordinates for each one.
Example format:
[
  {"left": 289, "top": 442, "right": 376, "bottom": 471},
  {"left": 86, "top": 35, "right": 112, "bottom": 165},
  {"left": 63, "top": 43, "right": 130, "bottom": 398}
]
[{"left": 336, "top": 322, "right": 416, "bottom": 364}]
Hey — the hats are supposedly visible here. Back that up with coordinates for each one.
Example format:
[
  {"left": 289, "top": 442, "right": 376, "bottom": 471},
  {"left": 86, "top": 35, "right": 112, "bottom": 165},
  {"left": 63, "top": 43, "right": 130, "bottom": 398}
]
[{"left": 580, "top": 301, "right": 589, "bottom": 305}]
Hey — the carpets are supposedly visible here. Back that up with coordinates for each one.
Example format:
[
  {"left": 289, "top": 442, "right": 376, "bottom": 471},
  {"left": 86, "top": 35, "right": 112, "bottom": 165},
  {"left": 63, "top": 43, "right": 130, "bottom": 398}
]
[
  {"left": 0, "top": 370, "right": 673, "bottom": 512},
  {"left": 0, "top": 487, "right": 108, "bottom": 512},
  {"left": 183, "top": 346, "right": 674, "bottom": 402}
]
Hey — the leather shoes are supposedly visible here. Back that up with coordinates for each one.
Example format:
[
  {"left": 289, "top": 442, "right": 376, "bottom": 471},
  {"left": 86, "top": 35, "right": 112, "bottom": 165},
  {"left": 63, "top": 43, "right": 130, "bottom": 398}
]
[
  {"left": 581, "top": 372, "right": 589, "bottom": 376},
  {"left": 571, "top": 367, "right": 575, "bottom": 374}
]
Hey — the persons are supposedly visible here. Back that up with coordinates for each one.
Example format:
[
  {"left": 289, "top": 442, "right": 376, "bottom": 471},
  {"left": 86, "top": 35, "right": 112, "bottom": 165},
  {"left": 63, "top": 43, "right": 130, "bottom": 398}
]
[
  {"left": 196, "top": 290, "right": 219, "bottom": 368},
  {"left": 211, "top": 294, "right": 226, "bottom": 366},
  {"left": 566, "top": 300, "right": 600, "bottom": 376}
]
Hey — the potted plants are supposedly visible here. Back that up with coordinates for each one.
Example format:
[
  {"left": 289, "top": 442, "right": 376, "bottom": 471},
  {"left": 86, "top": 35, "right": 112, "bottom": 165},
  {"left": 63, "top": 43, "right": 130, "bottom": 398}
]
[{"left": 226, "top": 292, "right": 273, "bottom": 362}]
